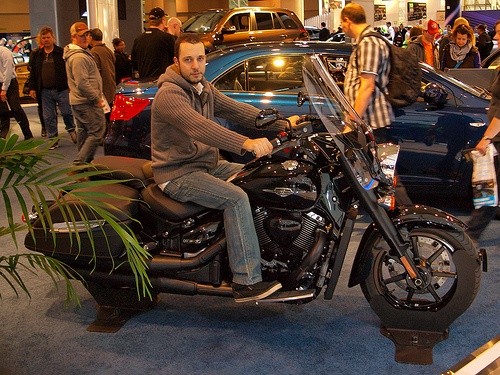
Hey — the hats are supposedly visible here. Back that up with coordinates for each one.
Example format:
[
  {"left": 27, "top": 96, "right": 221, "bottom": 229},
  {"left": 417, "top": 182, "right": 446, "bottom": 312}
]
[
  {"left": 476, "top": 25, "right": 487, "bottom": 30},
  {"left": 149, "top": 8, "right": 169, "bottom": 20},
  {"left": 69, "top": 22, "right": 92, "bottom": 38},
  {"left": 422, "top": 19, "right": 441, "bottom": 36}
]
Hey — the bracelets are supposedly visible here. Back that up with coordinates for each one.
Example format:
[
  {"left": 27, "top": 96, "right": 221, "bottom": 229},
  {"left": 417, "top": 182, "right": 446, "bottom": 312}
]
[{"left": 483, "top": 135, "right": 492, "bottom": 140}]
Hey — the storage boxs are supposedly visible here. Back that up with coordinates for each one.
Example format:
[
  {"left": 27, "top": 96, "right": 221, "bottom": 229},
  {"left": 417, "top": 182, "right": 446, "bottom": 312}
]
[{"left": 25, "top": 181, "right": 143, "bottom": 262}]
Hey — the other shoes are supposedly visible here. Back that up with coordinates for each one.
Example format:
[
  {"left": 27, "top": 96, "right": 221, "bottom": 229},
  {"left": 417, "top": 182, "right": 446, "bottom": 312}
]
[
  {"left": 69, "top": 171, "right": 88, "bottom": 183},
  {"left": 232, "top": 279, "right": 283, "bottom": 302},
  {"left": 68, "top": 129, "right": 77, "bottom": 144},
  {"left": 49, "top": 143, "right": 59, "bottom": 149},
  {"left": 41, "top": 127, "right": 47, "bottom": 137}
]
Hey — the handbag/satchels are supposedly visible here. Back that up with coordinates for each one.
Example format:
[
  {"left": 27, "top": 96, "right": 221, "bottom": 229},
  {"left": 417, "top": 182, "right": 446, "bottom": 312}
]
[{"left": 468, "top": 142, "right": 499, "bottom": 209}]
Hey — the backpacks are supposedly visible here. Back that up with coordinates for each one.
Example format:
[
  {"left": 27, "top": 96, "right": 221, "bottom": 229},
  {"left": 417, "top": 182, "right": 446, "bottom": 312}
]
[{"left": 355, "top": 32, "right": 423, "bottom": 108}]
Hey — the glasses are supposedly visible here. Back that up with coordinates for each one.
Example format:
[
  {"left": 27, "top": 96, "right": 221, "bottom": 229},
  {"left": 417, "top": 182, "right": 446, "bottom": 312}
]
[{"left": 177, "top": 25, "right": 184, "bottom": 33}]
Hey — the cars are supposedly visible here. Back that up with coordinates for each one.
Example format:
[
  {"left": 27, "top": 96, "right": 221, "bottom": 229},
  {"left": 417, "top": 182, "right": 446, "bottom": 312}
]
[
  {"left": 103, "top": 40, "right": 500, "bottom": 217},
  {"left": 180, "top": 5, "right": 355, "bottom": 54},
  {"left": 10, "top": 35, "right": 39, "bottom": 104}
]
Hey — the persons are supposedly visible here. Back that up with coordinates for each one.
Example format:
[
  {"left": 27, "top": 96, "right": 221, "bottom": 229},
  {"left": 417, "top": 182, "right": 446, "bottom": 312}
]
[
  {"left": 319, "top": 22, "right": 342, "bottom": 41},
  {"left": 383, "top": 17, "right": 494, "bottom": 69},
  {"left": 0, "top": 46, "right": 34, "bottom": 140},
  {"left": 152, "top": 34, "right": 301, "bottom": 303},
  {"left": 466, "top": 21, "right": 500, "bottom": 240},
  {"left": 339, "top": 4, "right": 396, "bottom": 142},
  {"left": 0, "top": 38, "right": 32, "bottom": 55},
  {"left": 28, "top": 27, "right": 77, "bottom": 150},
  {"left": 63, "top": 22, "right": 106, "bottom": 181},
  {"left": 130, "top": 7, "right": 182, "bottom": 82},
  {"left": 87, "top": 28, "right": 133, "bottom": 109}
]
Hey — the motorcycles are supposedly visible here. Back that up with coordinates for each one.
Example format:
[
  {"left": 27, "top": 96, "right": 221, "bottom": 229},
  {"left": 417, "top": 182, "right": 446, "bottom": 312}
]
[{"left": 20, "top": 51, "right": 482, "bottom": 332}]
